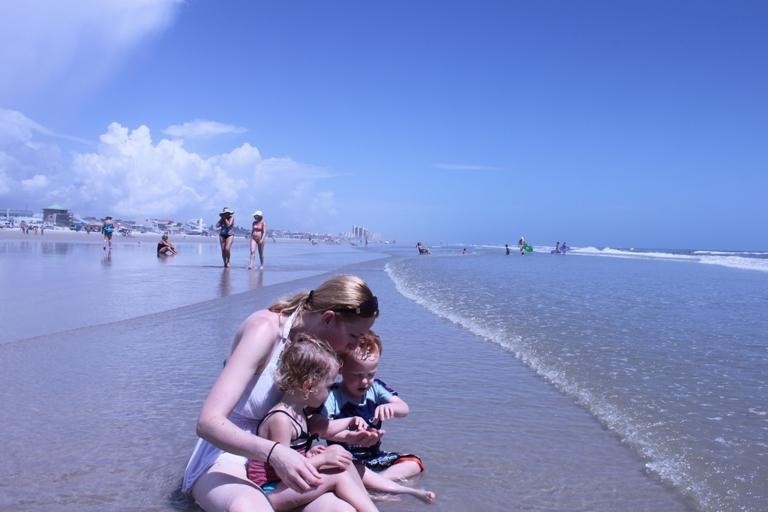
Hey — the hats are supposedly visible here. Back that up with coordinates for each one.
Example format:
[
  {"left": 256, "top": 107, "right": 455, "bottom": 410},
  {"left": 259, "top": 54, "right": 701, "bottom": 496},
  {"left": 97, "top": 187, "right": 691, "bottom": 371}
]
[
  {"left": 252, "top": 211, "right": 263, "bottom": 216},
  {"left": 219, "top": 207, "right": 234, "bottom": 216}
]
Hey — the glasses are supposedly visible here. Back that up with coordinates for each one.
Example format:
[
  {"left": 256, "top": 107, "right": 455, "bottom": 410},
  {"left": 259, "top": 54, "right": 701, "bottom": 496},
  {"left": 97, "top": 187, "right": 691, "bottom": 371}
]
[{"left": 321, "top": 296, "right": 378, "bottom": 318}]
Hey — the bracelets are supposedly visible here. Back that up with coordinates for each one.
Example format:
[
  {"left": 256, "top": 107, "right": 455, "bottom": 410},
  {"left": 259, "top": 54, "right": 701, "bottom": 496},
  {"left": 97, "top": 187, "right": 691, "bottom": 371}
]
[{"left": 267, "top": 441, "right": 280, "bottom": 465}]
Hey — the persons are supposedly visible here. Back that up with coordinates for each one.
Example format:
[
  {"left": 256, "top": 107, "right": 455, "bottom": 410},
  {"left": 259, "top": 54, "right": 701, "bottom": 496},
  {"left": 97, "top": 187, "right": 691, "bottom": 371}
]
[
  {"left": 247, "top": 330, "right": 385, "bottom": 512},
  {"left": 504, "top": 243, "right": 510, "bottom": 256},
  {"left": 561, "top": 241, "right": 567, "bottom": 256},
  {"left": 462, "top": 248, "right": 466, "bottom": 254},
  {"left": 102, "top": 248, "right": 113, "bottom": 268},
  {"left": 219, "top": 265, "right": 233, "bottom": 299},
  {"left": 312, "top": 330, "right": 438, "bottom": 505},
  {"left": 156, "top": 234, "right": 177, "bottom": 256},
  {"left": 214, "top": 206, "right": 235, "bottom": 267},
  {"left": 180, "top": 271, "right": 382, "bottom": 512},
  {"left": 248, "top": 268, "right": 264, "bottom": 291},
  {"left": 248, "top": 210, "right": 266, "bottom": 270},
  {"left": 554, "top": 241, "right": 559, "bottom": 255},
  {"left": 101, "top": 216, "right": 116, "bottom": 250},
  {"left": 20, "top": 220, "right": 46, "bottom": 235},
  {"left": 518, "top": 236, "right": 525, "bottom": 256},
  {"left": 416, "top": 242, "right": 431, "bottom": 255}
]
